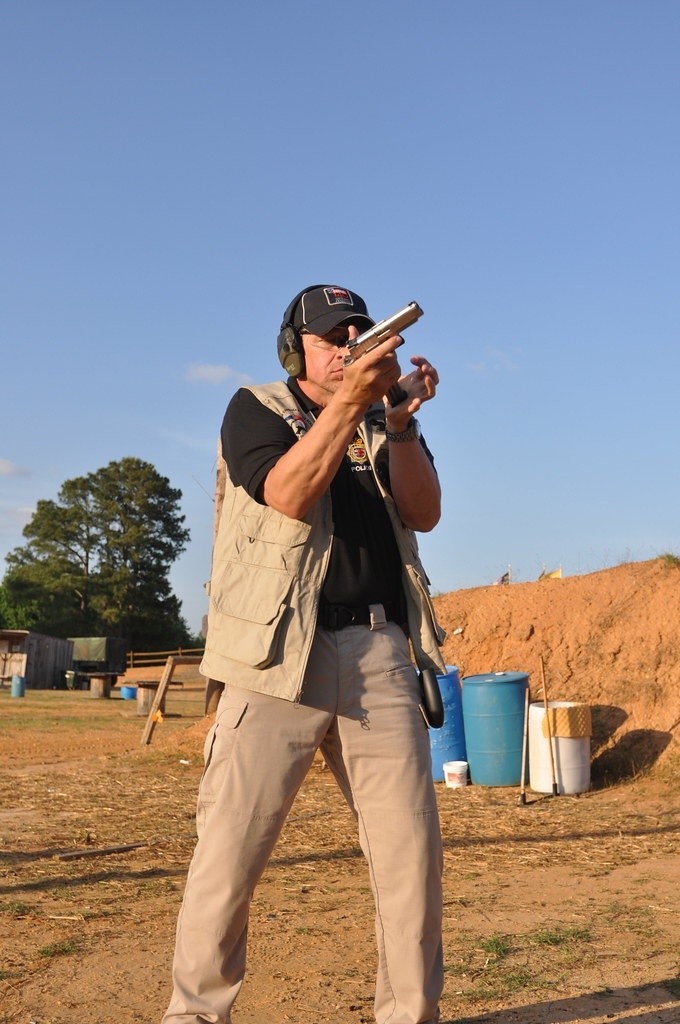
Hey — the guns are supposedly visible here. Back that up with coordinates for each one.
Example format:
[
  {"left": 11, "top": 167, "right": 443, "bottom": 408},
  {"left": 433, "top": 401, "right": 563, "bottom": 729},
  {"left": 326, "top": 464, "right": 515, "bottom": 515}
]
[{"left": 344, "top": 299, "right": 425, "bottom": 410}]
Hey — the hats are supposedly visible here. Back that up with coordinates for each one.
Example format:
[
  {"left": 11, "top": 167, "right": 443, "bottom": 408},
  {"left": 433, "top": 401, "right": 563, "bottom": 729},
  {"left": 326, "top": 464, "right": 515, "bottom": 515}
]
[{"left": 293, "top": 285, "right": 376, "bottom": 336}]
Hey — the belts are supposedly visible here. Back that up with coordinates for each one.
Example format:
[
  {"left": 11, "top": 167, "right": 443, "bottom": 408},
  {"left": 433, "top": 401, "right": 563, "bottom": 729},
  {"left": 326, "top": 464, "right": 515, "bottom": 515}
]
[{"left": 317, "top": 604, "right": 398, "bottom": 631}]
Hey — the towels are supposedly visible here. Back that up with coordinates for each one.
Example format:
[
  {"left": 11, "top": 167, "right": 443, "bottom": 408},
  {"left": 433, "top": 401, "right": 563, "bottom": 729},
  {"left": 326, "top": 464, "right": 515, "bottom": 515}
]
[{"left": 541, "top": 703, "right": 593, "bottom": 737}]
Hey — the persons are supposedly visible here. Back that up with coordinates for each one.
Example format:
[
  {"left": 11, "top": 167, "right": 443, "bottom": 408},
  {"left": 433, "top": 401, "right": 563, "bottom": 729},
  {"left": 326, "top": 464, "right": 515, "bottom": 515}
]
[{"left": 161, "top": 282, "right": 448, "bottom": 1024}]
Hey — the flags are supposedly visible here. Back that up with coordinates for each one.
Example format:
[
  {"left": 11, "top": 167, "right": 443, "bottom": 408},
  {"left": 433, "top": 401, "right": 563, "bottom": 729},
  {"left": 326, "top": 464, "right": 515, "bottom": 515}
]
[
  {"left": 494, "top": 571, "right": 510, "bottom": 584},
  {"left": 538, "top": 569, "right": 561, "bottom": 580}
]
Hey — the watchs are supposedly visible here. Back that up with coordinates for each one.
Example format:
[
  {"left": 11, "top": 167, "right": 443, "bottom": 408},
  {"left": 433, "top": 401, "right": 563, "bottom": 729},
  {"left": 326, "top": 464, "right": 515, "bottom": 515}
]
[{"left": 385, "top": 419, "right": 422, "bottom": 442}]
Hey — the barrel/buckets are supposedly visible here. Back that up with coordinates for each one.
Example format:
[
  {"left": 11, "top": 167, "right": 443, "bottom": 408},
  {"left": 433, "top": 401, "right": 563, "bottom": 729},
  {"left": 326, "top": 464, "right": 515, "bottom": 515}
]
[
  {"left": 413, "top": 665, "right": 468, "bottom": 783},
  {"left": 529, "top": 700, "right": 592, "bottom": 794},
  {"left": 12, "top": 675, "right": 26, "bottom": 698},
  {"left": 120, "top": 687, "right": 136, "bottom": 700},
  {"left": 444, "top": 761, "right": 468, "bottom": 788},
  {"left": 462, "top": 672, "right": 532, "bottom": 785}
]
